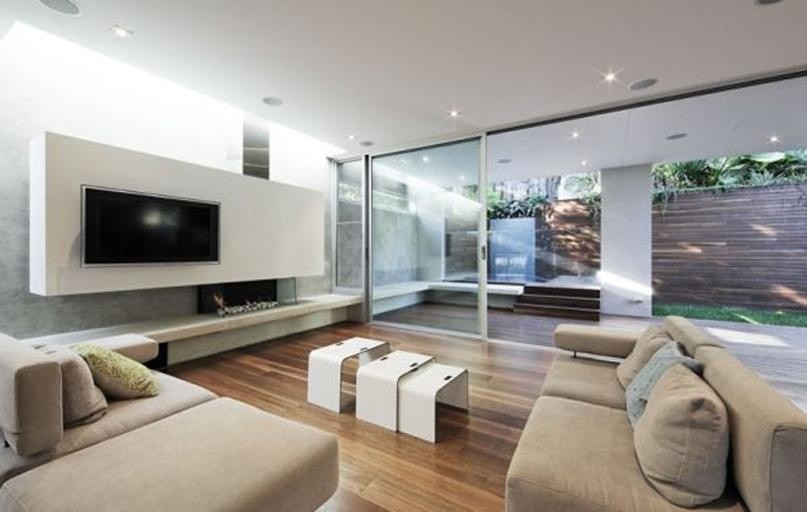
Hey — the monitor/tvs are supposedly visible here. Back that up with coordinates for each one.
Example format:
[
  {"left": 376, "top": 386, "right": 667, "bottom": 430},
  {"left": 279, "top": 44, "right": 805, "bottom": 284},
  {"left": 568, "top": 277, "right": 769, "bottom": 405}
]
[{"left": 80, "top": 182, "right": 222, "bottom": 268}]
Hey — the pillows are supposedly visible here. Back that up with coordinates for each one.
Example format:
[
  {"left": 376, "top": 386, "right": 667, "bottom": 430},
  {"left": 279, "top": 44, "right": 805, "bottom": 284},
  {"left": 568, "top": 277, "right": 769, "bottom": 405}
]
[
  {"left": 625, "top": 342, "right": 703, "bottom": 431},
  {"left": 616, "top": 325, "right": 673, "bottom": 390},
  {"left": 65, "top": 343, "right": 160, "bottom": 398},
  {"left": 30, "top": 344, "right": 109, "bottom": 427},
  {"left": 633, "top": 364, "right": 730, "bottom": 508}
]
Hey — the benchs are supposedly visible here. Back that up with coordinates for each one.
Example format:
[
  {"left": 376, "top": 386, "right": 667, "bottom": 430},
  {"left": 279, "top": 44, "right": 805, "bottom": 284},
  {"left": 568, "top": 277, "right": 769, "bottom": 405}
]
[{"left": 0, "top": 327, "right": 339, "bottom": 512}]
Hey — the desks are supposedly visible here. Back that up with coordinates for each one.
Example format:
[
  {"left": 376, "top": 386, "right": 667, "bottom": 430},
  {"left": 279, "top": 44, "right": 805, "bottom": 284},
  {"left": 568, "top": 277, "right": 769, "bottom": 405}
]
[{"left": 131, "top": 292, "right": 363, "bottom": 365}]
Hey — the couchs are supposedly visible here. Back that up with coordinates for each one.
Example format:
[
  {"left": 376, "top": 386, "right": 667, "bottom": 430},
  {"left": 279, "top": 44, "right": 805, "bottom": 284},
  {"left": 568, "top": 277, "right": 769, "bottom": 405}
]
[{"left": 504, "top": 315, "right": 807, "bottom": 512}]
